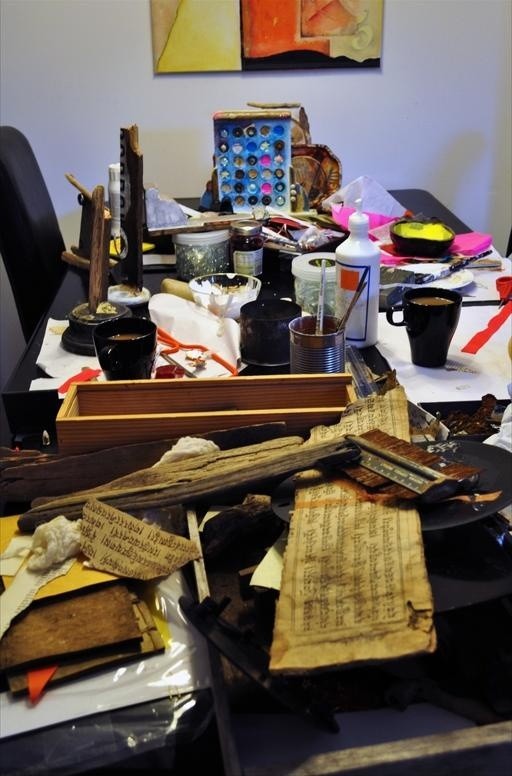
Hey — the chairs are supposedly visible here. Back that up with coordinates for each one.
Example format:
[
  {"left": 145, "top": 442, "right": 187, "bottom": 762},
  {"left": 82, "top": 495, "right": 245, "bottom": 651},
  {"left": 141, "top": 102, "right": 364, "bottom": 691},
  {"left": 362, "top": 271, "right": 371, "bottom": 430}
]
[{"left": 1, "top": 125, "right": 69, "bottom": 343}]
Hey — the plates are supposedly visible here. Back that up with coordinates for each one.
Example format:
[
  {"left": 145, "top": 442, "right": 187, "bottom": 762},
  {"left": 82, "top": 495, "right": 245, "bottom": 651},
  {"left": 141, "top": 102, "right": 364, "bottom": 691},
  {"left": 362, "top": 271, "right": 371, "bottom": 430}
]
[
  {"left": 271, "top": 441, "right": 512, "bottom": 538},
  {"left": 393, "top": 261, "right": 475, "bottom": 291}
]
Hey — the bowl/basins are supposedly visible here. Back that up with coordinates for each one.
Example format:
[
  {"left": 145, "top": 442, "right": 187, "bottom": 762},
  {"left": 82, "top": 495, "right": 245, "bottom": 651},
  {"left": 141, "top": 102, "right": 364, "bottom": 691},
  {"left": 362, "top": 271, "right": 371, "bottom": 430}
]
[
  {"left": 389, "top": 219, "right": 455, "bottom": 255},
  {"left": 187, "top": 273, "right": 263, "bottom": 317}
]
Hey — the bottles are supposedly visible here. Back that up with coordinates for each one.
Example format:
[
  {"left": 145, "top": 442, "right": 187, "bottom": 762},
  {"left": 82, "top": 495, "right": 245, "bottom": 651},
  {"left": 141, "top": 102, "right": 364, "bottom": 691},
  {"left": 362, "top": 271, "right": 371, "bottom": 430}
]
[
  {"left": 106, "top": 162, "right": 122, "bottom": 236},
  {"left": 290, "top": 250, "right": 335, "bottom": 317},
  {"left": 172, "top": 228, "right": 232, "bottom": 282},
  {"left": 230, "top": 219, "right": 265, "bottom": 289}
]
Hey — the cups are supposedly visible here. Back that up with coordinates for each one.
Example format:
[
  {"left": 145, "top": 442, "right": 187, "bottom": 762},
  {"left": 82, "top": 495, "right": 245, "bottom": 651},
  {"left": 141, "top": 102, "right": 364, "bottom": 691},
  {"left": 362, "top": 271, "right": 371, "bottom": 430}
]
[
  {"left": 386, "top": 287, "right": 463, "bottom": 368},
  {"left": 287, "top": 313, "right": 346, "bottom": 374},
  {"left": 94, "top": 318, "right": 157, "bottom": 380},
  {"left": 239, "top": 298, "right": 300, "bottom": 368}
]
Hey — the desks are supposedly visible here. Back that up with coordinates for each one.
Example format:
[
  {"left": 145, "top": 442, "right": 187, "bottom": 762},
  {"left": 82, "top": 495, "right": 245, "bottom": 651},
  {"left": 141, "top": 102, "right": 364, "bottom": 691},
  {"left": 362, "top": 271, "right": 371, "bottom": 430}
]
[{"left": 0, "top": 184, "right": 478, "bottom": 452}]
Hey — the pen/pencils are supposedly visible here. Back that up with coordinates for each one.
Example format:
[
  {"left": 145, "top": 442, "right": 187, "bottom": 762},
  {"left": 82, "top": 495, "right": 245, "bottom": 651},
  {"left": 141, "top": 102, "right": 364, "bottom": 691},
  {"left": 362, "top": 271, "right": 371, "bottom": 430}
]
[{"left": 316, "top": 259, "right": 368, "bottom": 335}]
